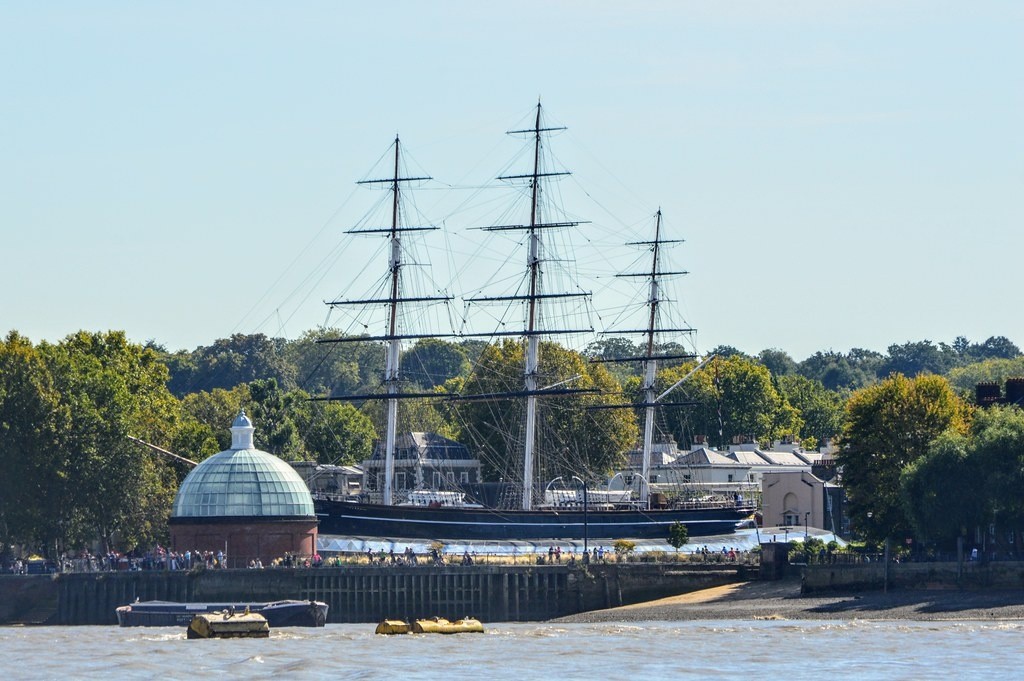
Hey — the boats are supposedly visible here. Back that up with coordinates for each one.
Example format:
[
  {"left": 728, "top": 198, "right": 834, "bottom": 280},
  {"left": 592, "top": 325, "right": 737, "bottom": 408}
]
[{"left": 115, "top": 596, "right": 329, "bottom": 628}]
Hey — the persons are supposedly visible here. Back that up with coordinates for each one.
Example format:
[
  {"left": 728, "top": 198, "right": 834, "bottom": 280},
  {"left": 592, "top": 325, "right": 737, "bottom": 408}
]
[
  {"left": 246, "top": 551, "right": 323, "bottom": 569},
  {"left": 614, "top": 547, "right": 635, "bottom": 563},
  {"left": 390, "top": 549, "right": 395, "bottom": 563},
  {"left": 430, "top": 549, "right": 473, "bottom": 566},
  {"left": 536, "top": 552, "right": 547, "bottom": 564},
  {"left": 333, "top": 555, "right": 341, "bottom": 567},
  {"left": 598, "top": 546, "right": 605, "bottom": 563},
  {"left": 819, "top": 543, "right": 1019, "bottom": 562},
  {"left": 368, "top": 548, "right": 373, "bottom": 564},
  {"left": 593, "top": 548, "right": 598, "bottom": 563},
  {"left": 555, "top": 547, "right": 561, "bottom": 565},
  {"left": 396, "top": 546, "right": 416, "bottom": 566},
  {"left": 707, "top": 493, "right": 743, "bottom": 506},
  {"left": 695, "top": 546, "right": 749, "bottom": 563},
  {"left": 379, "top": 549, "right": 386, "bottom": 562},
  {"left": 547, "top": 546, "right": 555, "bottom": 564},
  {"left": 13, "top": 548, "right": 228, "bottom": 575}
]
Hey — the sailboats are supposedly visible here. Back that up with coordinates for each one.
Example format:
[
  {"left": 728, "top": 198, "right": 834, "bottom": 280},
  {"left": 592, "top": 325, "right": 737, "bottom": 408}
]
[{"left": 288, "top": 93, "right": 764, "bottom": 538}]
